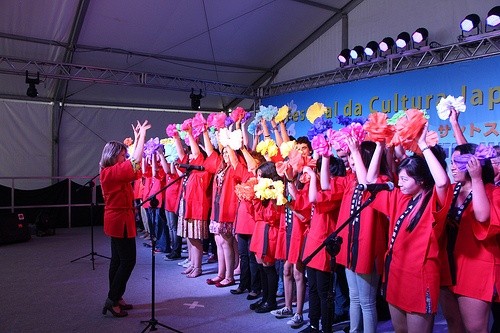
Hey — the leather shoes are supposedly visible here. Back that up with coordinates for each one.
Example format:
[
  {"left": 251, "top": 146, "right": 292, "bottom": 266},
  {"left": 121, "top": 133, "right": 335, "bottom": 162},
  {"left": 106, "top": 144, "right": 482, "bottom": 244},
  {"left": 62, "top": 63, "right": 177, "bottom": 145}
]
[
  {"left": 298, "top": 324, "right": 320, "bottom": 333},
  {"left": 250, "top": 299, "right": 277, "bottom": 313}
]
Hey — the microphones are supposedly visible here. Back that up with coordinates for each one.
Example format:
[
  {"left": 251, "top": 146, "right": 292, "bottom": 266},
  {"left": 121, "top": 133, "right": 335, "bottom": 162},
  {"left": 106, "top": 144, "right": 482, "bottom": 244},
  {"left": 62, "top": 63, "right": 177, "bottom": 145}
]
[
  {"left": 178, "top": 164, "right": 204, "bottom": 171},
  {"left": 358, "top": 182, "right": 394, "bottom": 192}
]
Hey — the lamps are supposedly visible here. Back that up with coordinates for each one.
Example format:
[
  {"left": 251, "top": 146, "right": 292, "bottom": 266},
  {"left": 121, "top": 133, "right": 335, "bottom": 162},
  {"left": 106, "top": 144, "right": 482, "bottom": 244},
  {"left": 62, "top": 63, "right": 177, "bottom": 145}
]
[
  {"left": 337, "top": 4, "right": 500, "bottom": 70},
  {"left": 188, "top": 88, "right": 204, "bottom": 112},
  {"left": 24, "top": 70, "right": 43, "bottom": 98}
]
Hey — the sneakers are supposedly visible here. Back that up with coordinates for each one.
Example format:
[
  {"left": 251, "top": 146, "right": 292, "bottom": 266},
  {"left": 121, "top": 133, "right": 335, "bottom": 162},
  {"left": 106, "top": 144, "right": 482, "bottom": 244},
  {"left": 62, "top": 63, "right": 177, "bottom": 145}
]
[
  {"left": 270, "top": 306, "right": 293, "bottom": 317},
  {"left": 287, "top": 312, "right": 303, "bottom": 326}
]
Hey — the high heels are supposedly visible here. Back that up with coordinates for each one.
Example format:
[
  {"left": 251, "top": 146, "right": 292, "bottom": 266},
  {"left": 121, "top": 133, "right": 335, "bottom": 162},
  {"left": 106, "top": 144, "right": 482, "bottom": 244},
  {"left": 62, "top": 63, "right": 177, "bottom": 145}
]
[{"left": 102, "top": 303, "right": 133, "bottom": 317}]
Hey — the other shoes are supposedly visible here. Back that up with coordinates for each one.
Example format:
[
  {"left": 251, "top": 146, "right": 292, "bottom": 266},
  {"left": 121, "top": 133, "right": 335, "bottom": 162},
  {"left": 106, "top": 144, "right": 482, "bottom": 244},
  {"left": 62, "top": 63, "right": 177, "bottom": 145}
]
[
  {"left": 140, "top": 232, "right": 240, "bottom": 278},
  {"left": 207, "top": 276, "right": 236, "bottom": 287},
  {"left": 231, "top": 288, "right": 260, "bottom": 299}
]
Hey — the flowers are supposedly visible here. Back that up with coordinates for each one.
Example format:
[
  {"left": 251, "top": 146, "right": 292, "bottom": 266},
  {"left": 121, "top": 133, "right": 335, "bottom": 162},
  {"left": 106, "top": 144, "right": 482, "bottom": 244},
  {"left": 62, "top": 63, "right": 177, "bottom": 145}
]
[{"left": 124, "top": 94, "right": 497, "bottom": 205}]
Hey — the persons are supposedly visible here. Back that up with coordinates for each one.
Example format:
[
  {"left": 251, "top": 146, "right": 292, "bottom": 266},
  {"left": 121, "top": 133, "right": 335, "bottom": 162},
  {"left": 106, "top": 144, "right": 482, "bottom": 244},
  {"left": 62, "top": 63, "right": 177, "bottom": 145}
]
[
  {"left": 99, "top": 120, "right": 151, "bottom": 317},
  {"left": 134, "top": 107, "right": 500, "bottom": 333}
]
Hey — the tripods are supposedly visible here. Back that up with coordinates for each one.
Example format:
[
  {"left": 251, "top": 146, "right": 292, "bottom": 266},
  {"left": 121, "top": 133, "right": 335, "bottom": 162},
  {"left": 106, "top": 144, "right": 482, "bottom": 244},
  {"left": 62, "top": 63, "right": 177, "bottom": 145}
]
[
  {"left": 139, "top": 172, "right": 196, "bottom": 332},
  {"left": 69, "top": 173, "right": 111, "bottom": 270}
]
